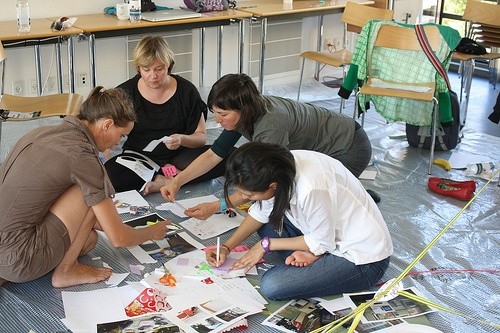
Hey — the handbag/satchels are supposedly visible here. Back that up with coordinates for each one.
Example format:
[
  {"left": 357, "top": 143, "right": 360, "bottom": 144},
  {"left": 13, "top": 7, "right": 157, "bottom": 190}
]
[{"left": 405, "top": 90, "right": 460, "bottom": 150}]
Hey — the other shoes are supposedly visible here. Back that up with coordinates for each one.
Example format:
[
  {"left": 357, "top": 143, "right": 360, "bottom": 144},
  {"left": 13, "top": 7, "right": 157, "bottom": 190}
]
[{"left": 428, "top": 176, "right": 476, "bottom": 201}]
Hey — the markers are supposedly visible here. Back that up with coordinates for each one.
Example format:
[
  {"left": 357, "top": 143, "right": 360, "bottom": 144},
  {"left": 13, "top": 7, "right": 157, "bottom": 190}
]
[{"left": 146, "top": 221, "right": 180, "bottom": 230}]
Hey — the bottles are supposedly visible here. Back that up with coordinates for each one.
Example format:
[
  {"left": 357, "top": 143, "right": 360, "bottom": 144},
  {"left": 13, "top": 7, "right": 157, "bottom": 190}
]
[
  {"left": 130, "top": 0, "right": 142, "bottom": 23},
  {"left": 16, "top": 0, "right": 31, "bottom": 33}
]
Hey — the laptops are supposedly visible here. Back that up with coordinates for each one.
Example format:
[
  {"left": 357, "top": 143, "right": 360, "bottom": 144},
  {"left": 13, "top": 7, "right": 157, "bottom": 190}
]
[{"left": 140, "top": 9, "right": 202, "bottom": 22}]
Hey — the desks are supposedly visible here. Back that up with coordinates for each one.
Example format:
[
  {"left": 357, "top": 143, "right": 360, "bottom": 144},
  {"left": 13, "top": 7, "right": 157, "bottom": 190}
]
[
  {"left": 49, "top": 9, "right": 253, "bottom": 91},
  {"left": 233, "top": 0, "right": 375, "bottom": 94},
  {"left": 0, "top": 19, "right": 83, "bottom": 95}
]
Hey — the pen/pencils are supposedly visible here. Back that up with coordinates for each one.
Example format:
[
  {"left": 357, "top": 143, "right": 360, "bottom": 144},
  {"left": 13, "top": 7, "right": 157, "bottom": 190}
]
[
  {"left": 236, "top": 4, "right": 258, "bottom": 10},
  {"left": 216, "top": 235, "right": 221, "bottom": 269}
]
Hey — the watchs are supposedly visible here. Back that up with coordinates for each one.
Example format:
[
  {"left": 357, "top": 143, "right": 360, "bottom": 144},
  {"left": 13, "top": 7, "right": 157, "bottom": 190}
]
[{"left": 261, "top": 236, "right": 272, "bottom": 254}]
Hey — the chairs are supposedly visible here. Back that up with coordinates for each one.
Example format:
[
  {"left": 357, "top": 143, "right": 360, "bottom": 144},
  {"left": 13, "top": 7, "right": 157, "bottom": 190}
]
[
  {"left": 0, "top": 41, "right": 83, "bottom": 142},
  {"left": 297, "top": 0, "right": 500, "bottom": 174}
]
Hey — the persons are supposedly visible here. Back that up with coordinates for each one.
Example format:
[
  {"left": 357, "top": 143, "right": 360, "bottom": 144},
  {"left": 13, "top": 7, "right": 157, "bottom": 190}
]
[
  {"left": 0, "top": 86, "right": 170, "bottom": 287},
  {"left": 103, "top": 35, "right": 238, "bottom": 196},
  {"left": 160, "top": 73, "right": 380, "bottom": 220},
  {"left": 206, "top": 142, "right": 394, "bottom": 301}
]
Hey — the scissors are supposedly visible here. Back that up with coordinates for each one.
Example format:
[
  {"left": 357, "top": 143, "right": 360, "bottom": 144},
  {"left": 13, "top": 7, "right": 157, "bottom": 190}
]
[
  {"left": 239, "top": 204, "right": 247, "bottom": 208},
  {"left": 159, "top": 260, "right": 177, "bottom": 287}
]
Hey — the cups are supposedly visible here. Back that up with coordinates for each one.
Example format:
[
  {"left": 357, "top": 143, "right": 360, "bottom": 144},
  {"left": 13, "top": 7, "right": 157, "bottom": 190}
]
[{"left": 116, "top": 3, "right": 130, "bottom": 21}]
